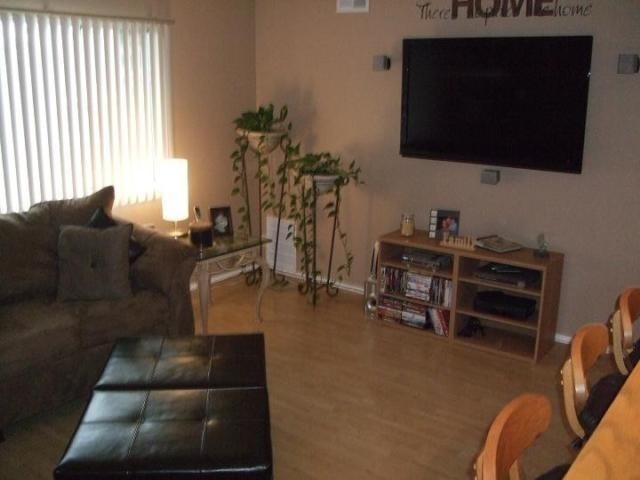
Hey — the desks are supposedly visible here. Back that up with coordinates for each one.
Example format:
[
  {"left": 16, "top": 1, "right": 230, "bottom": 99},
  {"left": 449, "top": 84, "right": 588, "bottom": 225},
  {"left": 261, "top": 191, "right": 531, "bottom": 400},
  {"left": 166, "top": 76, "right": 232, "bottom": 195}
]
[
  {"left": 562, "top": 362, "right": 639, "bottom": 480},
  {"left": 161, "top": 223, "right": 275, "bottom": 335}
]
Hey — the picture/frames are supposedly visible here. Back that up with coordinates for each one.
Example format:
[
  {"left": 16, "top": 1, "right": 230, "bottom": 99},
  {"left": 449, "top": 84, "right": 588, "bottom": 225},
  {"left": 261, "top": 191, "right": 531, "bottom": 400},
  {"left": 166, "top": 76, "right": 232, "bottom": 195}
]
[
  {"left": 209, "top": 206, "right": 233, "bottom": 234},
  {"left": 429, "top": 208, "right": 460, "bottom": 240}
]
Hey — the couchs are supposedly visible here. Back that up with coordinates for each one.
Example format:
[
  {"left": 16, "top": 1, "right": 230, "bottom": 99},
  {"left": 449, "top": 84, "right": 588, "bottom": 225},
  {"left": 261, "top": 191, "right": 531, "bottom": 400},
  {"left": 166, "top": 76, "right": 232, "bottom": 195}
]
[{"left": 0, "top": 199, "right": 193, "bottom": 438}]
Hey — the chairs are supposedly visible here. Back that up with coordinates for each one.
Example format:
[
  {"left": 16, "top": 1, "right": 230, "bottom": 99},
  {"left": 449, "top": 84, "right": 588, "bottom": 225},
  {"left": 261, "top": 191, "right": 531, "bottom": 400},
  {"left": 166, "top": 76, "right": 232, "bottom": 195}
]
[
  {"left": 474, "top": 391, "right": 573, "bottom": 480},
  {"left": 557, "top": 319, "right": 631, "bottom": 447},
  {"left": 605, "top": 283, "right": 639, "bottom": 378}
]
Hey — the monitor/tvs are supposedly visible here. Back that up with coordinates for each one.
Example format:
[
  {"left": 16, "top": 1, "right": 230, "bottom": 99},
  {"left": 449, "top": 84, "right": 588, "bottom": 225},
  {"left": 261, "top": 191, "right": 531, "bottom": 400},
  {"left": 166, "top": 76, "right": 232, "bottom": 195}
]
[{"left": 401, "top": 35, "right": 593, "bottom": 175}]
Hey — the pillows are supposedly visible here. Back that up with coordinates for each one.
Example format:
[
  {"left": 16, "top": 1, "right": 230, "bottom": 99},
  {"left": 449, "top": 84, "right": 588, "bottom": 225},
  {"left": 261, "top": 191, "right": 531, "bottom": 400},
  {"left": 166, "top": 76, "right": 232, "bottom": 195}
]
[
  {"left": 57, "top": 225, "right": 134, "bottom": 301},
  {"left": 1, "top": 213, "right": 79, "bottom": 304},
  {"left": 30, "top": 185, "right": 116, "bottom": 226},
  {"left": 84, "top": 206, "right": 149, "bottom": 264}
]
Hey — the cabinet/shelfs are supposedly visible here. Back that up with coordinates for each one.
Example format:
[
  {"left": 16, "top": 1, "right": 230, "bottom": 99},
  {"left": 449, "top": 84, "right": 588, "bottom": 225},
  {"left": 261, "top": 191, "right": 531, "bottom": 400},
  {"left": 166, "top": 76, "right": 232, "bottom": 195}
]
[{"left": 374, "top": 226, "right": 565, "bottom": 361}]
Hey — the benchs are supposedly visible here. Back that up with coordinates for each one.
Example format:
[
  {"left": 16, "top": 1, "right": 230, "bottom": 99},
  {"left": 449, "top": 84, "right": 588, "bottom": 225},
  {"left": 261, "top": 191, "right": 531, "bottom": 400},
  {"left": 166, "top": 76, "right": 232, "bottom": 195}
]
[{"left": 54, "top": 331, "right": 276, "bottom": 480}]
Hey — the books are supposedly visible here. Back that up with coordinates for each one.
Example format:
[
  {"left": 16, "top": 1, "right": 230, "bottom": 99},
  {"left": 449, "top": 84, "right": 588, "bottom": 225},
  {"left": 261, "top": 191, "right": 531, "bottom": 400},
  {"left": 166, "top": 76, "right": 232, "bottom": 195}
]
[
  {"left": 476, "top": 232, "right": 525, "bottom": 256},
  {"left": 374, "top": 245, "right": 456, "bottom": 339}
]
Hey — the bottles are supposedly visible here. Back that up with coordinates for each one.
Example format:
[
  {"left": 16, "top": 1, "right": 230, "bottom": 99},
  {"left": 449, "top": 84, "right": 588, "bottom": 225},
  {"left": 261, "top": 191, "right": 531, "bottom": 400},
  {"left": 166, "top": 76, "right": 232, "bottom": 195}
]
[{"left": 400, "top": 213, "right": 415, "bottom": 237}]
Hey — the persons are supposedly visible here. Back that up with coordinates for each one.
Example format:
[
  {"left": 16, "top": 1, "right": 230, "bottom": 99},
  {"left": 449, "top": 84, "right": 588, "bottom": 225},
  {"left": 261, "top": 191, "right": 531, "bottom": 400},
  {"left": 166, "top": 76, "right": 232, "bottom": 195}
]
[
  {"left": 213, "top": 211, "right": 228, "bottom": 233},
  {"left": 438, "top": 216, "right": 458, "bottom": 234}
]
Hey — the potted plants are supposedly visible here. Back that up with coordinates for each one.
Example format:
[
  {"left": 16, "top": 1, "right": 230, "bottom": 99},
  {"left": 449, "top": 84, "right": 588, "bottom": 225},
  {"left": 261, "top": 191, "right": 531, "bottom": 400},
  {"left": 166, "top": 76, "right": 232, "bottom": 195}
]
[
  {"left": 283, "top": 149, "right": 366, "bottom": 285},
  {"left": 229, "top": 100, "right": 297, "bottom": 284}
]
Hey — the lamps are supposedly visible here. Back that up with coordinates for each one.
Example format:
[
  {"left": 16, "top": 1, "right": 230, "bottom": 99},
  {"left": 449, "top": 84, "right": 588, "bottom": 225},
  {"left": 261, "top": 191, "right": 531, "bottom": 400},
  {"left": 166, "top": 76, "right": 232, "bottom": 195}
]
[{"left": 160, "top": 155, "right": 191, "bottom": 237}]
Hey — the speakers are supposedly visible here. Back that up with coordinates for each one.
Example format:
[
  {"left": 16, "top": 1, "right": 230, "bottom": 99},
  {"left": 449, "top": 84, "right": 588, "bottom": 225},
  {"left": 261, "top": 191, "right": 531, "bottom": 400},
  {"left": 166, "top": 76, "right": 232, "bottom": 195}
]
[{"left": 364, "top": 279, "right": 380, "bottom": 318}]
